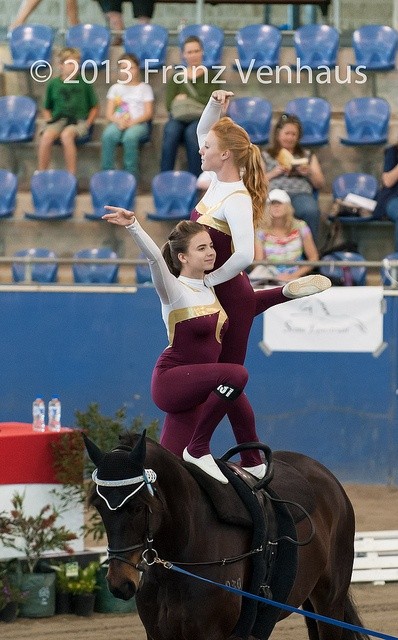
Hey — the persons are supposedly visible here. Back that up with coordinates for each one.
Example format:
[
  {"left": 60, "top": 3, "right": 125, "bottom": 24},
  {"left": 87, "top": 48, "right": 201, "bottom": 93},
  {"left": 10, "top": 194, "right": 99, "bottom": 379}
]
[
  {"left": 35, "top": 47, "right": 99, "bottom": 173},
  {"left": 254, "top": 189, "right": 319, "bottom": 280},
  {"left": 258, "top": 112, "right": 325, "bottom": 249},
  {"left": 100, "top": 53, "right": 154, "bottom": 175},
  {"left": 189, "top": 89, "right": 332, "bottom": 480},
  {"left": 160, "top": 36, "right": 220, "bottom": 178},
  {"left": 101, "top": 205, "right": 248, "bottom": 485},
  {"left": 381, "top": 141, "right": 398, "bottom": 253}
]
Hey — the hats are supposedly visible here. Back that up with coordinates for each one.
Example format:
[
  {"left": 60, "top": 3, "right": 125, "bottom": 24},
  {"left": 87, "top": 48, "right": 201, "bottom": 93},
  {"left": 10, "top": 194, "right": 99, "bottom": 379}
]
[{"left": 266, "top": 189, "right": 290, "bottom": 204}]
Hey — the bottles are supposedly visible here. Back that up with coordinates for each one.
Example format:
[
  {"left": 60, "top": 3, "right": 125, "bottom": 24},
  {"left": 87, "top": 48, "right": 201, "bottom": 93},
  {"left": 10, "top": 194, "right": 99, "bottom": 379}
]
[
  {"left": 31, "top": 396, "right": 46, "bottom": 433},
  {"left": 47, "top": 394, "right": 62, "bottom": 433}
]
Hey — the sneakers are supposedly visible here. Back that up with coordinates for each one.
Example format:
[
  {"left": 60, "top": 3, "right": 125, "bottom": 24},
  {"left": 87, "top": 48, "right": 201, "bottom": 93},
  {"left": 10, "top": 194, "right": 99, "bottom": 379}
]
[
  {"left": 282, "top": 274, "right": 332, "bottom": 298},
  {"left": 182, "top": 447, "right": 228, "bottom": 485},
  {"left": 241, "top": 464, "right": 266, "bottom": 479}
]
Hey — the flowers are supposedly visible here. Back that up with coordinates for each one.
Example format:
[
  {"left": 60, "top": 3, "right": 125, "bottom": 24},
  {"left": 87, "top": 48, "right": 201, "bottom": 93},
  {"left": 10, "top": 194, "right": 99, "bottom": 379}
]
[
  {"left": 59, "top": 561, "right": 102, "bottom": 595},
  {"left": 0, "top": 487, "right": 80, "bottom": 573},
  {"left": 0, "top": 572, "right": 30, "bottom": 608}
]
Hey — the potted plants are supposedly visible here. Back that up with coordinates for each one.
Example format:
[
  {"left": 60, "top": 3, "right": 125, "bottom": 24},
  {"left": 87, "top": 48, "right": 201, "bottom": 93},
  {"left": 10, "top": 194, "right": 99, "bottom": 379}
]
[{"left": 50, "top": 401, "right": 162, "bottom": 614}]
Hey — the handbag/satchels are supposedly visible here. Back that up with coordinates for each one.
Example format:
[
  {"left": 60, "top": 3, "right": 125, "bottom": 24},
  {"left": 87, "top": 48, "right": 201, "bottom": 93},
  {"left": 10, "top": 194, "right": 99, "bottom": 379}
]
[{"left": 170, "top": 71, "right": 206, "bottom": 121}]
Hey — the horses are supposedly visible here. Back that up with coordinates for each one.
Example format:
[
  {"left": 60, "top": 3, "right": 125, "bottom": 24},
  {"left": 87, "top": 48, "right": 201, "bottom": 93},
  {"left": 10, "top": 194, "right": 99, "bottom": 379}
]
[{"left": 80, "top": 428, "right": 371, "bottom": 640}]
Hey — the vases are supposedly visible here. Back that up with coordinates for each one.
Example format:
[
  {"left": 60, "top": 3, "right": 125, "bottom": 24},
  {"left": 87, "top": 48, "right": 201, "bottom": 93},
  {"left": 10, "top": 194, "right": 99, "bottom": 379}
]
[
  {"left": 1, "top": 603, "right": 20, "bottom": 621},
  {"left": 8, "top": 573, "right": 58, "bottom": 619},
  {"left": 56, "top": 591, "right": 99, "bottom": 616}
]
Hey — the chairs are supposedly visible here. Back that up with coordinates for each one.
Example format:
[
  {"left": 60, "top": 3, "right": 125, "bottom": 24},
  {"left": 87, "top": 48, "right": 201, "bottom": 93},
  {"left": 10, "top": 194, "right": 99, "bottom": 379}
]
[
  {"left": 121, "top": 19, "right": 169, "bottom": 71},
  {"left": 5, "top": 22, "right": 53, "bottom": 72},
  {"left": 293, "top": 22, "right": 341, "bottom": 71},
  {"left": 382, "top": 252, "right": 397, "bottom": 292},
  {"left": 350, "top": 24, "right": 398, "bottom": 70},
  {"left": 224, "top": 95, "right": 272, "bottom": 147},
  {"left": 145, "top": 169, "right": 205, "bottom": 221},
  {"left": 8, "top": 246, "right": 60, "bottom": 287},
  {"left": 20, "top": 167, "right": 78, "bottom": 222},
  {"left": 82, "top": 169, "right": 139, "bottom": 225},
  {"left": 319, "top": 250, "right": 367, "bottom": 286},
  {"left": 177, "top": 22, "right": 224, "bottom": 71},
  {"left": 0, "top": 93, "right": 39, "bottom": 144},
  {"left": 284, "top": 96, "right": 329, "bottom": 148},
  {"left": 339, "top": 95, "right": 391, "bottom": 147},
  {"left": 64, "top": 20, "right": 112, "bottom": 71},
  {"left": 135, "top": 251, "right": 155, "bottom": 283},
  {"left": 331, "top": 172, "right": 377, "bottom": 225},
  {"left": 231, "top": 22, "right": 281, "bottom": 72},
  {"left": 0, "top": 166, "right": 17, "bottom": 220},
  {"left": 71, "top": 246, "right": 119, "bottom": 285}
]
